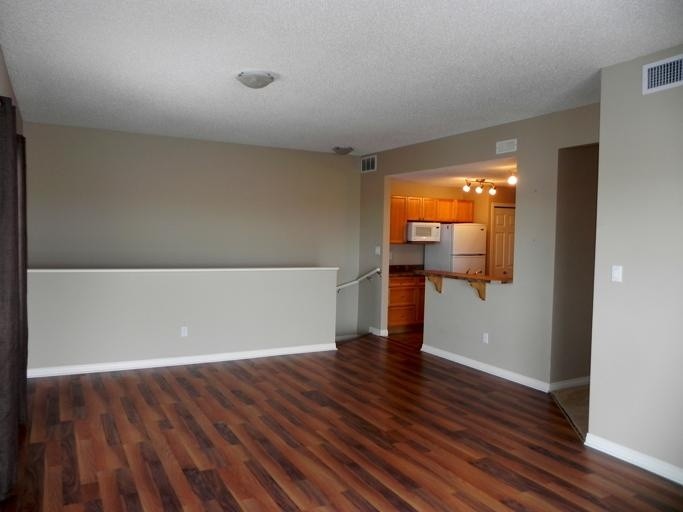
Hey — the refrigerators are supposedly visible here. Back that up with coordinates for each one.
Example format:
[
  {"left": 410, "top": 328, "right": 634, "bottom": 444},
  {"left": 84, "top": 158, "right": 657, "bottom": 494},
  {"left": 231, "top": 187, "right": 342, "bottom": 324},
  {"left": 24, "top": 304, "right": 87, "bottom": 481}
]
[{"left": 423, "top": 222, "right": 486, "bottom": 278}]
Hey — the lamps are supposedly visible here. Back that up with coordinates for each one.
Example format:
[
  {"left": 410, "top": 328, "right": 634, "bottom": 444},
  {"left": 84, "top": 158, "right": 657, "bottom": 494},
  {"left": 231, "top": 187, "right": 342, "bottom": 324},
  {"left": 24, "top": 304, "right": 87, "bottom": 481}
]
[{"left": 462, "top": 178, "right": 497, "bottom": 196}]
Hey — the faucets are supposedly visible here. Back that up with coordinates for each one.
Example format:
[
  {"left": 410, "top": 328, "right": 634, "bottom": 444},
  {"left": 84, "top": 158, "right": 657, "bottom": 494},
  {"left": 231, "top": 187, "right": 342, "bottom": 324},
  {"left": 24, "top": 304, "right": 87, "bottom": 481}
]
[{"left": 475, "top": 270, "right": 483, "bottom": 275}]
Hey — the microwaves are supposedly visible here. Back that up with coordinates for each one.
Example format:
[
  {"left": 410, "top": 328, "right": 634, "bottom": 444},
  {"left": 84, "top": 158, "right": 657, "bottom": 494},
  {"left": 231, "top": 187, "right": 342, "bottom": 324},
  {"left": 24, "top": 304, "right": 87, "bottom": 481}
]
[{"left": 406, "top": 221, "right": 441, "bottom": 242}]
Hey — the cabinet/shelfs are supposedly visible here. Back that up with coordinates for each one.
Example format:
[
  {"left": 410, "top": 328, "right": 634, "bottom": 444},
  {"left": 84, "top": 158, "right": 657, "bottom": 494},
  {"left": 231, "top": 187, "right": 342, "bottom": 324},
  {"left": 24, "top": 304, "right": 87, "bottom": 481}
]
[
  {"left": 407, "top": 197, "right": 475, "bottom": 223},
  {"left": 390, "top": 195, "right": 407, "bottom": 244},
  {"left": 388, "top": 274, "right": 424, "bottom": 334}
]
